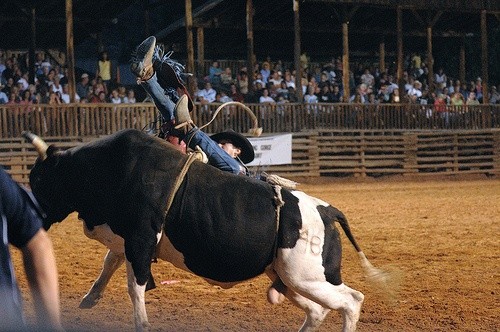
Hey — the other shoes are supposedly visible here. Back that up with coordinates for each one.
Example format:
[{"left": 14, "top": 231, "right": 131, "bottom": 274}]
[
  {"left": 132, "top": 35, "right": 156, "bottom": 77},
  {"left": 173, "top": 94, "right": 193, "bottom": 135}
]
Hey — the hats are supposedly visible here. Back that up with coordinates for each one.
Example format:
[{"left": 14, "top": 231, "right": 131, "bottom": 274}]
[{"left": 210, "top": 128, "right": 254, "bottom": 164}]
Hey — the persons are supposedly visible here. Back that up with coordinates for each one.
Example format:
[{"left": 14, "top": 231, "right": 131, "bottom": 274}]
[
  {"left": 450, "top": 91, "right": 466, "bottom": 130},
  {"left": 304, "top": 85, "right": 318, "bottom": 129},
  {"left": 276, "top": 92, "right": 290, "bottom": 132},
  {"left": 0, "top": 167, "right": 60, "bottom": 332},
  {"left": 392, "top": 88, "right": 402, "bottom": 129},
  {"left": 350, "top": 93, "right": 364, "bottom": 129},
  {"left": 0, "top": 52, "right": 500, "bottom": 138},
  {"left": 318, "top": 86, "right": 330, "bottom": 129},
  {"left": 7, "top": 92, "right": 18, "bottom": 137},
  {"left": 330, "top": 86, "right": 341, "bottom": 128},
  {"left": 131, "top": 36, "right": 255, "bottom": 177},
  {"left": 466, "top": 92, "right": 482, "bottom": 130},
  {"left": 200, "top": 80, "right": 216, "bottom": 133},
  {"left": 433, "top": 93, "right": 447, "bottom": 129},
  {"left": 216, "top": 83, "right": 235, "bottom": 132},
  {"left": 97, "top": 51, "right": 111, "bottom": 89},
  {"left": 229, "top": 83, "right": 245, "bottom": 132},
  {"left": 260, "top": 89, "right": 276, "bottom": 132},
  {"left": 20, "top": 90, "right": 35, "bottom": 137},
  {"left": 365, "top": 93, "right": 379, "bottom": 128}
]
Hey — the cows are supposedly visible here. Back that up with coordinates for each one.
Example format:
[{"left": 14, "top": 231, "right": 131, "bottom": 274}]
[{"left": 18, "top": 124, "right": 394, "bottom": 332}]
